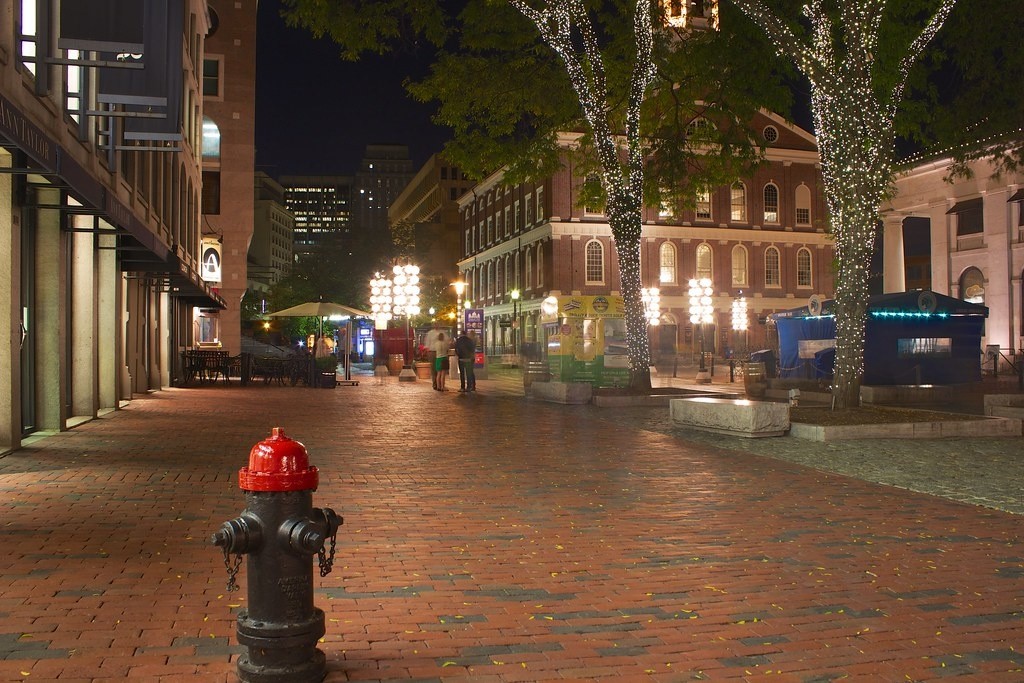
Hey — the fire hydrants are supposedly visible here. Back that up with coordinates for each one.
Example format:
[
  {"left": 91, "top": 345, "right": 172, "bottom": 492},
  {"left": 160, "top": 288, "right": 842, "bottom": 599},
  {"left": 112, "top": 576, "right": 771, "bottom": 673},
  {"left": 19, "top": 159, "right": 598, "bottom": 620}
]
[{"left": 211, "top": 426, "right": 343, "bottom": 683}]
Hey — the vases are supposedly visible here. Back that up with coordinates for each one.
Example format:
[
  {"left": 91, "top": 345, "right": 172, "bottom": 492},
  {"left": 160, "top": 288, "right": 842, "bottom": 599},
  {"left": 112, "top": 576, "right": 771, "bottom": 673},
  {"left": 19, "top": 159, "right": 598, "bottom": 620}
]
[{"left": 414, "top": 363, "right": 431, "bottom": 379}]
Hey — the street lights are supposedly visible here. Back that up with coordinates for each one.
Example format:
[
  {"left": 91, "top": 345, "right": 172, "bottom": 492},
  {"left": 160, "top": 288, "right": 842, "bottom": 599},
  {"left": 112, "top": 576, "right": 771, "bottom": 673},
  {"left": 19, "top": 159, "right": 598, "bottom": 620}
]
[
  {"left": 731, "top": 289, "right": 748, "bottom": 340},
  {"left": 392, "top": 264, "right": 420, "bottom": 369},
  {"left": 511, "top": 290, "right": 520, "bottom": 354},
  {"left": 689, "top": 278, "right": 714, "bottom": 372},
  {"left": 640, "top": 288, "right": 660, "bottom": 367},
  {"left": 264, "top": 323, "right": 270, "bottom": 354},
  {"left": 366, "top": 268, "right": 392, "bottom": 365},
  {"left": 450, "top": 280, "right": 469, "bottom": 335}
]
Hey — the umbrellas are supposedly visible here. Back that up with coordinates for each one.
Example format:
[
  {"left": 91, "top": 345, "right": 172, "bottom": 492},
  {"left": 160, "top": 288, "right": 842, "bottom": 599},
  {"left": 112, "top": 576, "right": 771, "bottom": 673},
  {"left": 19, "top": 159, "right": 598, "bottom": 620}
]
[{"left": 269, "top": 295, "right": 372, "bottom": 360}]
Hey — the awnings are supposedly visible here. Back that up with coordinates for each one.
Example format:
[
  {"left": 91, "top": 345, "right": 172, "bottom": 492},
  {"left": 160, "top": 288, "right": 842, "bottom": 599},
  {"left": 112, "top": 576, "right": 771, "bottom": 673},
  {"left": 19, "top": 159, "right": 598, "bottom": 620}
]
[
  {"left": 1006, "top": 188, "right": 1024, "bottom": 202},
  {"left": 944, "top": 197, "right": 982, "bottom": 214}
]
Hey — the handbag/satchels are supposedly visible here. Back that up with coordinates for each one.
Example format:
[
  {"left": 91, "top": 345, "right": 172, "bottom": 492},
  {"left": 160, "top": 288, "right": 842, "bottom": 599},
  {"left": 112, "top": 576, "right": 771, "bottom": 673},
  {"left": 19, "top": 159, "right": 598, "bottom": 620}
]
[{"left": 442, "top": 360, "right": 448, "bottom": 368}]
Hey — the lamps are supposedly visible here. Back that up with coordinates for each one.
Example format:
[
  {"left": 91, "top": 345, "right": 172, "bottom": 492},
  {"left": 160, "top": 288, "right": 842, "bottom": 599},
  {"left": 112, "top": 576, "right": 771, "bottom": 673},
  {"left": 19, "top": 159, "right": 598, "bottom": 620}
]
[{"left": 201, "top": 228, "right": 225, "bottom": 245}]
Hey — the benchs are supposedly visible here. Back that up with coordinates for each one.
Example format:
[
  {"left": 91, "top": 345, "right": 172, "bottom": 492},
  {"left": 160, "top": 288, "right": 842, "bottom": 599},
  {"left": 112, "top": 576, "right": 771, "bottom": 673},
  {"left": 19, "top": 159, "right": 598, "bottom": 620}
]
[{"left": 185, "top": 349, "right": 230, "bottom": 384}]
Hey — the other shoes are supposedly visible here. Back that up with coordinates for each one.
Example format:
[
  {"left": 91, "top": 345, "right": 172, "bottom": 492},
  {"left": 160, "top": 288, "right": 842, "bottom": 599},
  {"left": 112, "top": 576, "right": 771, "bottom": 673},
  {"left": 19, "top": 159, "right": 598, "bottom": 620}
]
[
  {"left": 458, "top": 389, "right": 464, "bottom": 391},
  {"left": 441, "top": 388, "right": 449, "bottom": 391},
  {"left": 434, "top": 388, "right": 437, "bottom": 390},
  {"left": 466, "top": 389, "right": 471, "bottom": 391},
  {"left": 437, "top": 388, "right": 440, "bottom": 391}
]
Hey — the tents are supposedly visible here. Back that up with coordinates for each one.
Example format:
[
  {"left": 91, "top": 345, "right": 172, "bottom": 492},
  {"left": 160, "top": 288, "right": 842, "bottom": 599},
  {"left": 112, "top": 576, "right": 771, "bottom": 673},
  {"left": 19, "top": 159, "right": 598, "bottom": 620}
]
[{"left": 772, "top": 290, "right": 989, "bottom": 387}]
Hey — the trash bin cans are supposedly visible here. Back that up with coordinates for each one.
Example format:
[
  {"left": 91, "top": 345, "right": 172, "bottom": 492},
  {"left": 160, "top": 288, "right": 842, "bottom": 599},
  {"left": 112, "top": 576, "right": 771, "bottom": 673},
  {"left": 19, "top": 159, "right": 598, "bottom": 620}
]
[
  {"left": 744, "top": 362, "right": 768, "bottom": 399},
  {"left": 750, "top": 349, "right": 771, "bottom": 377},
  {"left": 388, "top": 354, "right": 404, "bottom": 376},
  {"left": 701, "top": 352, "right": 712, "bottom": 367},
  {"left": 523, "top": 360, "right": 549, "bottom": 397}
]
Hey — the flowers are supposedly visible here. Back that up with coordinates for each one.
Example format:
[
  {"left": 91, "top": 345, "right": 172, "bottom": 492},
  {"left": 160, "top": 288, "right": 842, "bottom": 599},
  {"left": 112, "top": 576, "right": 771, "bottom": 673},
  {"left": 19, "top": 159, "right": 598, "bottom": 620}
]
[{"left": 415, "top": 344, "right": 430, "bottom": 362}]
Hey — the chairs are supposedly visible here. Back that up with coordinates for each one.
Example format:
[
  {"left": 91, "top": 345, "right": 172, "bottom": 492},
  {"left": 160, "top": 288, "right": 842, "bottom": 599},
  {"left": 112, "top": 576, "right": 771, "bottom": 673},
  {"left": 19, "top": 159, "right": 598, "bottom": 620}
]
[{"left": 251, "top": 355, "right": 311, "bottom": 388}]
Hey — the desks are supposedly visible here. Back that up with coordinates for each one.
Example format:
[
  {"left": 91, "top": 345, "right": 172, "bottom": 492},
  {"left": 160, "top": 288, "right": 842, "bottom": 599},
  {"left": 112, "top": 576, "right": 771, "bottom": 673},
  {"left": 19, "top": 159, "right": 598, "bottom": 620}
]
[
  {"left": 263, "top": 357, "right": 292, "bottom": 386},
  {"left": 182, "top": 355, "right": 235, "bottom": 382}
]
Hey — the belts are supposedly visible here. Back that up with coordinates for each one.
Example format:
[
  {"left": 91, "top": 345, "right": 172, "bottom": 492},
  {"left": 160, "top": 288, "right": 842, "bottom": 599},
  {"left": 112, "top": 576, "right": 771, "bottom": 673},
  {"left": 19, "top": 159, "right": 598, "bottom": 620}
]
[{"left": 429, "top": 351, "right": 436, "bottom": 352}]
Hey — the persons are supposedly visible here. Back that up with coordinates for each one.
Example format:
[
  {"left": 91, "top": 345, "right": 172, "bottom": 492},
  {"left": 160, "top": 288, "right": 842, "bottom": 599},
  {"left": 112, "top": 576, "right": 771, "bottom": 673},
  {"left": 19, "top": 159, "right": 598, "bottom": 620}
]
[
  {"left": 469, "top": 330, "right": 478, "bottom": 391},
  {"left": 435, "top": 332, "right": 450, "bottom": 392},
  {"left": 424, "top": 321, "right": 449, "bottom": 390},
  {"left": 455, "top": 331, "right": 475, "bottom": 393}
]
